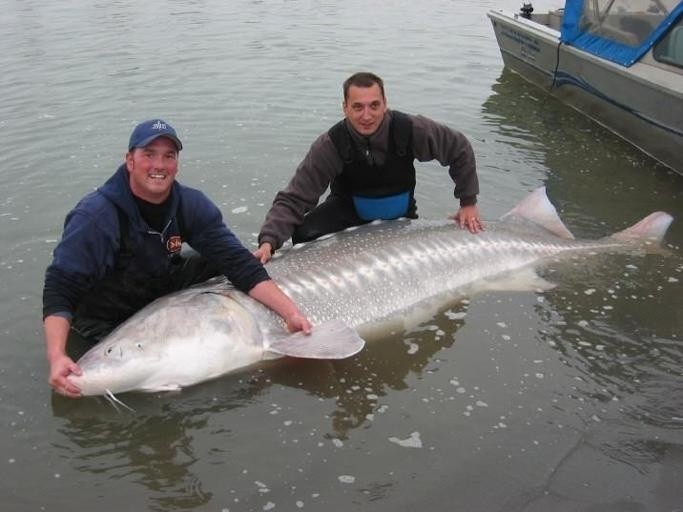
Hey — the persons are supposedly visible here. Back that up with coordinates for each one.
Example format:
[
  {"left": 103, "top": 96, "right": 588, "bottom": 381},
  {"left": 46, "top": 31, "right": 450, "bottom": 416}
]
[
  {"left": 41, "top": 118, "right": 313, "bottom": 399},
  {"left": 252, "top": 72, "right": 485, "bottom": 264}
]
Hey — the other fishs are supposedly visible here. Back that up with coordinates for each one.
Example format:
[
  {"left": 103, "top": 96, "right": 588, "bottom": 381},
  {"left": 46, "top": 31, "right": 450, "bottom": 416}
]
[{"left": 65, "top": 184, "right": 676, "bottom": 417}]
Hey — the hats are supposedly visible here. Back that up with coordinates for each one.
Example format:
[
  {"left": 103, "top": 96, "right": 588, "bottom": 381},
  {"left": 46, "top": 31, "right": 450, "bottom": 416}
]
[{"left": 129, "top": 118, "right": 183, "bottom": 155}]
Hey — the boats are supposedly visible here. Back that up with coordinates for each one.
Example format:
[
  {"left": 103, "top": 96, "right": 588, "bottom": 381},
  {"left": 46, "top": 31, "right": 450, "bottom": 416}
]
[
  {"left": 486, "top": 75, "right": 682, "bottom": 232},
  {"left": 486, "top": 0, "right": 682, "bottom": 182}
]
